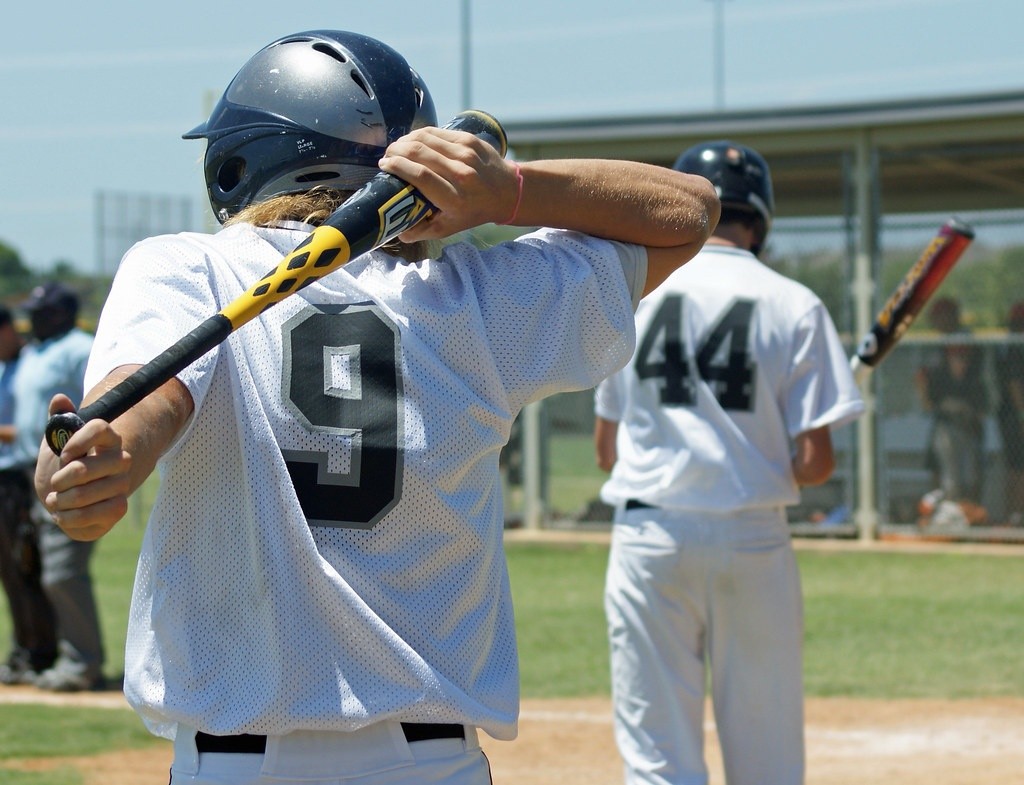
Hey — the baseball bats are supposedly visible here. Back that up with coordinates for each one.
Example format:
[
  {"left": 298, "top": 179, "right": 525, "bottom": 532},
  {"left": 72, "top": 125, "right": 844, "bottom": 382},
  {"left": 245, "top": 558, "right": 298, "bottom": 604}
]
[
  {"left": 42, "top": 109, "right": 509, "bottom": 459},
  {"left": 846, "top": 212, "right": 978, "bottom": 388}
]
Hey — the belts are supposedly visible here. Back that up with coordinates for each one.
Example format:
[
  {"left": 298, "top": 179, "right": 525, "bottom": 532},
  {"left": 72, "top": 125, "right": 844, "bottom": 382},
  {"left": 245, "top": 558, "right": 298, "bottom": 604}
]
[
  {"left": 624, "top": 499, "right": 652, "bottom": 511},
  {"left": 194, "top": 720, "right": 465, "bottom": 753}
]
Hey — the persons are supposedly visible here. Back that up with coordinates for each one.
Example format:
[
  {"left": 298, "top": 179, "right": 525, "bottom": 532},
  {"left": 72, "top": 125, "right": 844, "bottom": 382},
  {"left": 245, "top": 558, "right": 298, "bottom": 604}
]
[
  {"left": 803, "top": 299, "right": 1024, "bottom": 529},
  {"left": 593, "top": 141, "right": 867, "bottom": 784},
  {"left": 35, "top": 32, "right": 723, "bottom": 785},
  {"left": 0, "top": 280, "right": 108, "bottom": 691}
]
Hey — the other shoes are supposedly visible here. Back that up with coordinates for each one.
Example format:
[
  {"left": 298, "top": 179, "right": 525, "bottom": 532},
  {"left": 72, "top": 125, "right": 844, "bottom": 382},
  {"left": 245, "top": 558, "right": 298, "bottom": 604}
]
[
  {"left": 36, "top": 656, "right": 105, "bottom": 690},
  {"left": 0, "top": 649, "right": 38, "bottom": 685}
]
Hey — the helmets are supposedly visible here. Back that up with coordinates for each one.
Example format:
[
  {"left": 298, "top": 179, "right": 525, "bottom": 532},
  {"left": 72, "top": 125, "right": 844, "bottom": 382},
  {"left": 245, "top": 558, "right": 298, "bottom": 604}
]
[
  {"left": 675, "top": 140, "right": 773, "bottom": 257},
  {"left": 19, "top": 280, "right": 79, "bottom": 341},
  {"left": 182, "top": 30, "right": 438, "bottom": 225}
]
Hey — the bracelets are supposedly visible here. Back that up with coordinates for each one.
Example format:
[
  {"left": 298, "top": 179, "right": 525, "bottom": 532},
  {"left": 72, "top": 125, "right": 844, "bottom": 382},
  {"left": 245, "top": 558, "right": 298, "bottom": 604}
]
[{"left": 495, "top": 159, "right": 523, "bottom": 225}]
